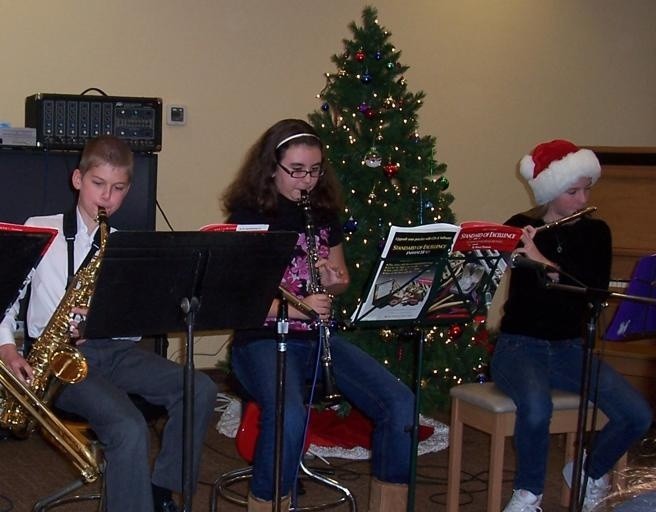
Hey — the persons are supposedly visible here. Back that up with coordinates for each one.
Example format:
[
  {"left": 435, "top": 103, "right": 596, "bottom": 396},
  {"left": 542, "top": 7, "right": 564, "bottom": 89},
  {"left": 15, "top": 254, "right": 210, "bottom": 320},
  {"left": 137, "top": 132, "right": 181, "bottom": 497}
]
[
  {"left": 223, "top": 121, "right": 417, "bottom": 512},
  {"left": 487, "top": 141, "right": 653, "bottom": 511},
  {"left": 1, "top": 135, "right": 216, "bottom": 511}
]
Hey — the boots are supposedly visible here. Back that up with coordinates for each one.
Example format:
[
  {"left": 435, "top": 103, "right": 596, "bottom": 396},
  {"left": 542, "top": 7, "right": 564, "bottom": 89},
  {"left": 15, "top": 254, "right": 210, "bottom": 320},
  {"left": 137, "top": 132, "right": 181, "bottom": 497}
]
[
  {"left": 244, "top": 490, "right": 294, "bottom": 511},
  {"left": 366, "top": 478, "right": 410, "bottom": 512}
]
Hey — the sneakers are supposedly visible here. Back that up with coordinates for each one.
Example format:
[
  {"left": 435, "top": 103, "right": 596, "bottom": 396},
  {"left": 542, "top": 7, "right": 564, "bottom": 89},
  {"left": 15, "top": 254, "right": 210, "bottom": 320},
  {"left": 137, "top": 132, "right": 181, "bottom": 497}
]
[
  {"left": 502, "top": 487, "right": 543, "bottom": 511},
  {"left": 561, "top": 449, "right": 612, "bottom": 508}
]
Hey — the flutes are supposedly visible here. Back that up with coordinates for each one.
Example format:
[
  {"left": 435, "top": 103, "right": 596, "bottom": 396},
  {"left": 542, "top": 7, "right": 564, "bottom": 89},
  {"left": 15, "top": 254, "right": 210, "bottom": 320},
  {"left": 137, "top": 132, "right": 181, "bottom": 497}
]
[{"left": 535, "top": 205, "right": 598, "bottom": 234}]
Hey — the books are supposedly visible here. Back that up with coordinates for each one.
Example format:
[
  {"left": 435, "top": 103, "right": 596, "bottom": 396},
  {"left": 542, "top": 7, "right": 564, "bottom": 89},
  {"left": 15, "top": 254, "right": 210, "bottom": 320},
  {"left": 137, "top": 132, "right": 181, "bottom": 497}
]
[
  {"left": 0, "top": 222, "right": 58, "bottom": 322},
  {"left": 194, "top": 223, "right": 269, "bottom": 232},
  {"left": 350, "top": 220, "right": 522, "bottom": 321}
]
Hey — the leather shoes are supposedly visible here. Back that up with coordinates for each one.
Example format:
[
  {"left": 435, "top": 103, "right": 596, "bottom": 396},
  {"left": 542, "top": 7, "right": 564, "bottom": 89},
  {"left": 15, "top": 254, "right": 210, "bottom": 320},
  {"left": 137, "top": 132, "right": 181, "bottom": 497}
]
[{"left": 149, "top": 498, "right": 181, "bottom": 512}]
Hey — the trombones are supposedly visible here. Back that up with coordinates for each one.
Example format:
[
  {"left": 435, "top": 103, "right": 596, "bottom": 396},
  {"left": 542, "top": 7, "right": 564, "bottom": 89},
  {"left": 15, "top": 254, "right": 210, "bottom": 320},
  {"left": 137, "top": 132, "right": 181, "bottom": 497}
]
[{"left": 0, "top": 356, "right": 107, "bottom": 485}]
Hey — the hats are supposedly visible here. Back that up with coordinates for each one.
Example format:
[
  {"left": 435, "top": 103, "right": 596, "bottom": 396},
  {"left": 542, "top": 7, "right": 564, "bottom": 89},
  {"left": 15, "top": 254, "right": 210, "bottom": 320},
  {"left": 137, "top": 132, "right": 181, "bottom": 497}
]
[{"left": 521, "top": 137, "right": 602, "bottom": 204}]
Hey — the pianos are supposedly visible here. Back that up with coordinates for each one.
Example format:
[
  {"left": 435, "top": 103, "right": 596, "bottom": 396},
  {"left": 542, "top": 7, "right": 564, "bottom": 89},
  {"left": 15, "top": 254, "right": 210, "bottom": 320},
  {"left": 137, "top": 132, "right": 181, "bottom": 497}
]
[{"left": 583, "top": 145, "right": 654, "bottom": 412}]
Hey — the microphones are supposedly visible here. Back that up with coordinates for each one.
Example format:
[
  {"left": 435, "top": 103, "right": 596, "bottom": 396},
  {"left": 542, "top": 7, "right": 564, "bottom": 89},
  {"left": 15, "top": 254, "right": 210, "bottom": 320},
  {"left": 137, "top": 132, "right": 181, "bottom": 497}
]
[
  {"left": 278, "top": 286, "right": 321, "bottom": 322},
  {"left": 508, "top": 253, "right": 552, "bottom": 278}
]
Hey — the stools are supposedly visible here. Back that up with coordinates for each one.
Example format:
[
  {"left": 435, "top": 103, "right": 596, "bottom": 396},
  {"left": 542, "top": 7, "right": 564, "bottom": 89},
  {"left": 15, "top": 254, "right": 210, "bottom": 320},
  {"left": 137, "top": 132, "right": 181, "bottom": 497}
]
[
  {"left": 209, "top": 368, "right": 357, "bottom": 511},
  {"left": 444, "top": 380, "right": 629, "bottom": 512},
  {"left": 30, "top": 394, "right": 165, "bottom": 511}
]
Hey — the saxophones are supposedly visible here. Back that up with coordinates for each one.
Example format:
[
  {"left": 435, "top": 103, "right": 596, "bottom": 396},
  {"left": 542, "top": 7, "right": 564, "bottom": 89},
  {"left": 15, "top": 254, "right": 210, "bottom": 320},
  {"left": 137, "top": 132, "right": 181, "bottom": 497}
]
[{"left": 0, "top": 206, "right": 111, "bottom": 443}]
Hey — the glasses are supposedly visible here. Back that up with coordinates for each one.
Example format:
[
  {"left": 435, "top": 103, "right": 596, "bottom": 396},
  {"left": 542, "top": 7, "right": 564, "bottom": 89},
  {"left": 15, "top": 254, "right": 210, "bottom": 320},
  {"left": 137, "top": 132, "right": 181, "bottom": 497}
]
[{"left": 275, "top": 160, "right": 323, "bottom": 178}]
